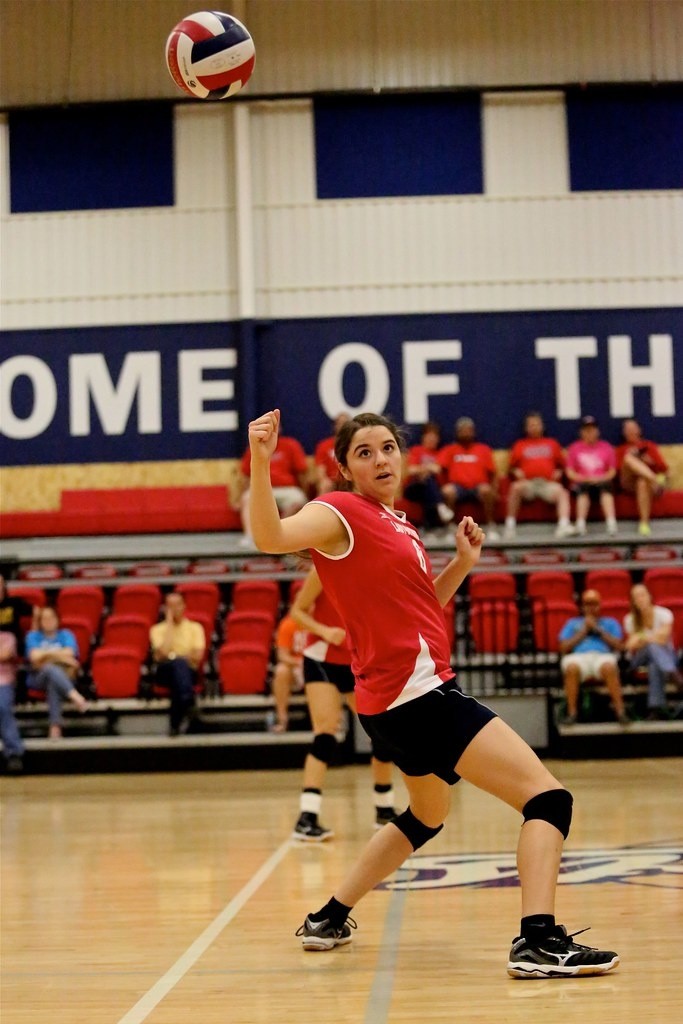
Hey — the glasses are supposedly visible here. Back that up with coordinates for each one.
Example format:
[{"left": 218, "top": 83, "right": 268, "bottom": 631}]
[{"left": 583, "top": 601, "right": 600, "bottom": 607}]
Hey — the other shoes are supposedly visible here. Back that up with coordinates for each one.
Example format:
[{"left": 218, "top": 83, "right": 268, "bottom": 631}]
[
  {"left": 505, "top": 528, "right": 516, "bottom": 539},
  {"left": 561, "top": 714, "right": 577, "bottom": 724},
  {"left": 658, "top": 474, "right": 667, "bottom": 488},
  {"left": 637, "top": 522, "right": 652, "bottom": 535},
  {"left": 554, "top": 525, "right": 576, "bottom": 538},
  {"left": 618, "top": 712, "right": 628, "bottom": 726},
  {"left": 576, "top": 525, "right": 587, "bottom": 536},
  {"left": 486, "top": 529, "right": 501, "bottom": 539},
  {"left": 607, "top": 519, "right": 617, "bottom": 535}
]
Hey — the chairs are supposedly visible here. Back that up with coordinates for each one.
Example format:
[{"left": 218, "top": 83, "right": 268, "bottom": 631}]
[{"left": 4, "top": 542, "right": 683, "bottom": 702}]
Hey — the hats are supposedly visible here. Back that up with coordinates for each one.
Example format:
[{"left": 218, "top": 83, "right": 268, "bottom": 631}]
[
  {"left": 456, "top": 417, "right": 475, "bottom": 431},
  {"left": 580, "top": 415, "right": 597, "bottom": 428}
]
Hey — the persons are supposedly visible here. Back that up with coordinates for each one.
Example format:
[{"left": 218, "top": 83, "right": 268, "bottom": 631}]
[
  {"left": 150, "top": 592, "right": 210, "bottom": 736},
  {"left": 291, "top": 568, "right": 399, "bottom": 842},
  {"left": 559, "top": 584, "right": 683, "bottom": 725},
  {"left": 232, "top": 413, "right": 502, "bottom": 541},
  {"left": 247, "top": 408, "right": 619, "bottom": 977},
  {"left": 503, "top": 412, "right": 669, "bottom": 538},
  {"left": 268, "top": 587, "right": 305, "bottom": 733},
  {"left": 0, "top": 571, "right": 92, "bottom": 759}
]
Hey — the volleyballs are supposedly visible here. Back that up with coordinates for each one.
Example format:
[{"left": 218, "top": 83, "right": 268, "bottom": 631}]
[{"left": 164, "top": 9, "right": 258, "bottom": 101}]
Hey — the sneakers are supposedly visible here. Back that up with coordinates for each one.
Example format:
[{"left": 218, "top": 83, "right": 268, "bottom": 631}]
[
  {"left": 293, "top": 818, "right": 333, "bottom": 841},
  {"left": 297, "top": 913, "right": 357, "bottom": 950},
  {"left": 505, "top": 925, "right": 620, "bottom": 977},
  {"left": 375, "top": 814, "right": 398, "bottom": 828}
]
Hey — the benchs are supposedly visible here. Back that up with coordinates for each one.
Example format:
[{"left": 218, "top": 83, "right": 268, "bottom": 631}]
[{"left": 0, "top": 478, "right": 683, "bottom": 541}]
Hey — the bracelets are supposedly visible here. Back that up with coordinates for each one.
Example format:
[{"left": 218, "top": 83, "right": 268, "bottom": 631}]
[
  {"left": 168, "top": 652, "right": 176, "bottom": 660},
  {"left": 637, "top": 631, "right": 642, "bottom": 640}
]
